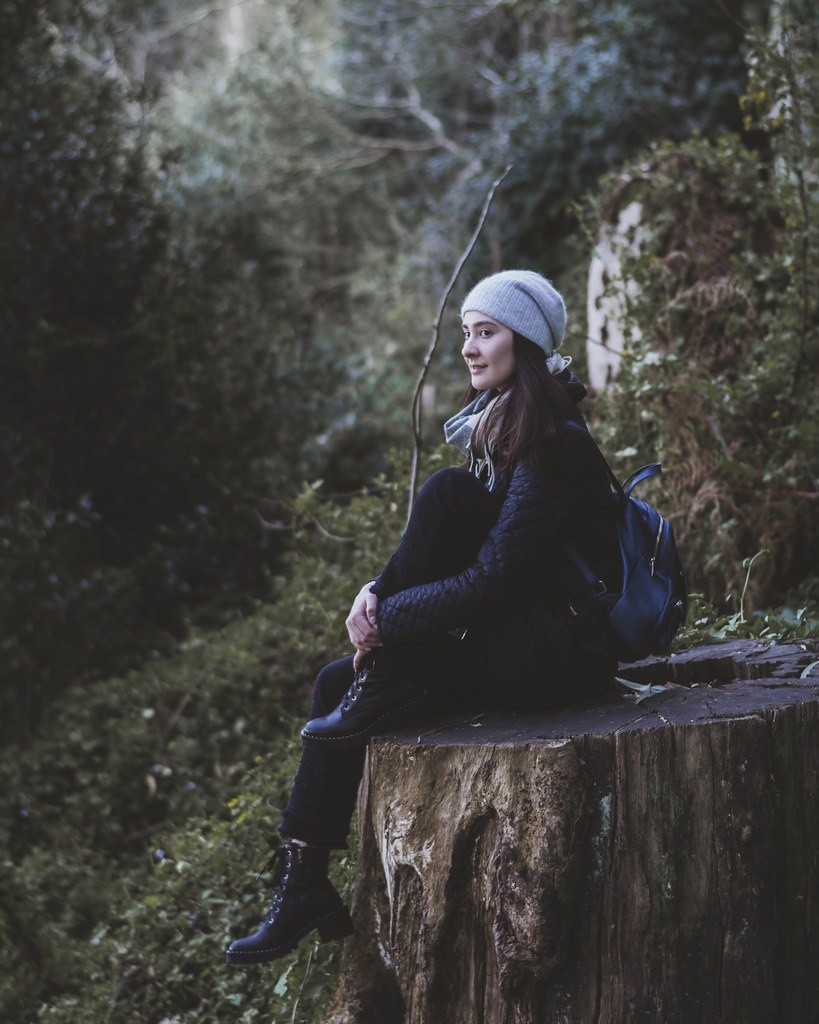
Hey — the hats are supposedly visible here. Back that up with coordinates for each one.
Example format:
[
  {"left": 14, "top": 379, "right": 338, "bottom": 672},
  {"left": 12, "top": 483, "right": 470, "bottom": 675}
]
[{"left": 460, "top": 270, "right": 567, "bottom": 358}]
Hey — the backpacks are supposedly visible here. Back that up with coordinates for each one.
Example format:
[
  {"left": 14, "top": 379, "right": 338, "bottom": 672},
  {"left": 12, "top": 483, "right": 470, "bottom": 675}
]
[{"left": 505, "top": 419, "right": 688, "bottom": 664}]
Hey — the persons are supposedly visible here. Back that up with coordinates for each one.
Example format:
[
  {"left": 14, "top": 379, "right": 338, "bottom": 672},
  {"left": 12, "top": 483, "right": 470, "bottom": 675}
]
[{"left": 225, "top": 269, "right": 687, "bottom": 963}]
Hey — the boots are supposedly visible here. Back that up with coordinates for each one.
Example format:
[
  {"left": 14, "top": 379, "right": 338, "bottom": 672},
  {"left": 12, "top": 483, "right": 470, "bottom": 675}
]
[
  {"left": 226, "top": 842, "right": 356, "bottom": 964},
  {"left": 301, "top": 646, "right": 428, "bottom": 746}
]
